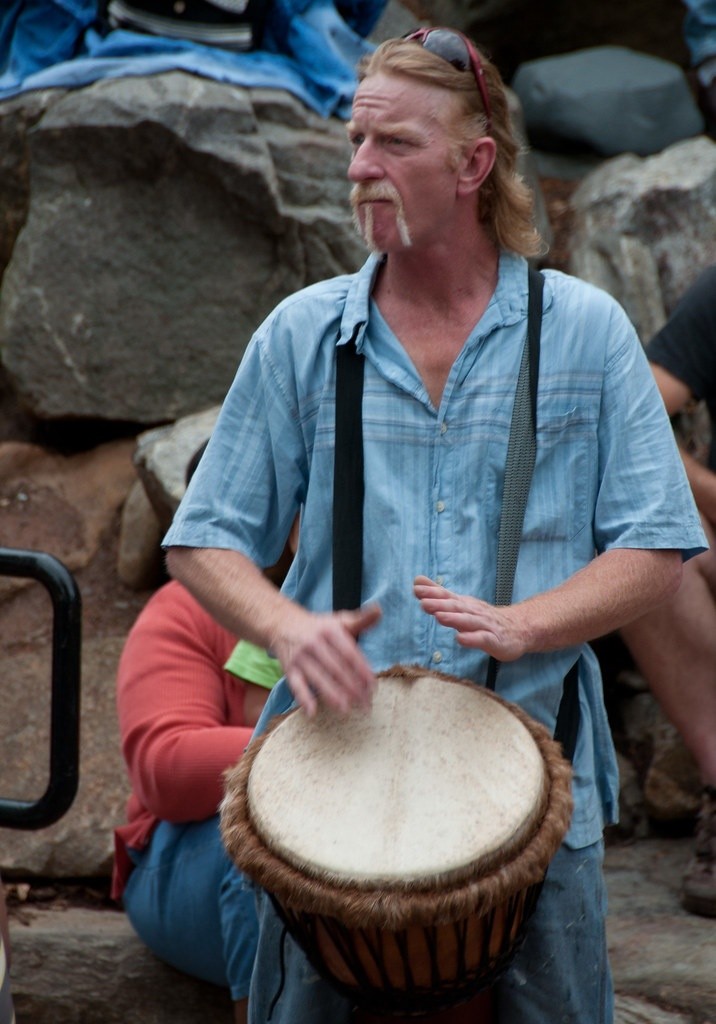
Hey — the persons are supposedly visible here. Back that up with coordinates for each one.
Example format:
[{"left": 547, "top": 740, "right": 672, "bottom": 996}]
[
  {"left": 158, "top": 29, "right": 712, "bottom": 1024},
  {"left": 108, "top": 439, "right": 309, "bottom": 1024},
  {"left": 616, "top": 264, "right": 716, "bottom": 913}
]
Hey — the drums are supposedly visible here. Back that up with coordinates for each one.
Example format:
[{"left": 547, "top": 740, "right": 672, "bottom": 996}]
[{"left": 249, "top": 670, "right": 551, "bottom": 1024}]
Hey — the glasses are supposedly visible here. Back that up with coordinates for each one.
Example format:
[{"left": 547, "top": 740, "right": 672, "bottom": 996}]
[{"left": 401, "top": 26, "right": 494, "bottom": 137}]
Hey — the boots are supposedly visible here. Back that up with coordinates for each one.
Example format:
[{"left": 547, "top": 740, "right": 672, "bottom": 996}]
[{"left": 682, "top": 784, "right": 716, "bottom": 919}]
